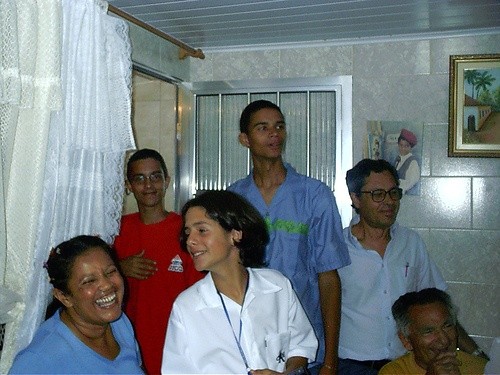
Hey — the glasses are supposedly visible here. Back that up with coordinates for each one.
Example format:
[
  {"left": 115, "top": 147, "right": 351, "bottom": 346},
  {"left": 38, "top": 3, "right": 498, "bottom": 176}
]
[
  {"left": 128, "top": 172, "right": 165, "bottom": 183},
  {"left": 360, "top": 188, "right": 402, "bottom": 202}
]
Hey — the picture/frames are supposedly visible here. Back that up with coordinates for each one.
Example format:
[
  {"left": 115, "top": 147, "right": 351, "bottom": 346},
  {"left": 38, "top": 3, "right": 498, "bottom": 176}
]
[{"left": 448, "top": 54, "right": 500, "bottom": 159}]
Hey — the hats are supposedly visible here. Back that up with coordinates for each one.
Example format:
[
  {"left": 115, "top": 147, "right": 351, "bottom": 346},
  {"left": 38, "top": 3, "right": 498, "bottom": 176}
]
[{"left": 400, "top": 128, "right": 417, "bottom": 145}]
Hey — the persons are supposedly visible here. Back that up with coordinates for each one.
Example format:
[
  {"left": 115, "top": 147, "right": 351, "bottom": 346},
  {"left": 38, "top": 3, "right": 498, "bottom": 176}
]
[
  {"left": 394, "top": 129, "right": 421, "bottom": 196},
  {"left": 6, "top": 234, "right": 145, "bottom": 375},
  {"left": 113, "top": 148, "right": 207, "bottom": 375},
  {"left": 336, "top": 159, "right": 490, "bottom": 374},
  {"left": 378, "top": 288, "right": 487, "bottom": 375},
  {"left": 160, "top": 190, "right": 318, "bottom": 375},
  {"left": 226, "top": 99, "right": 351, "bottom": 375}
]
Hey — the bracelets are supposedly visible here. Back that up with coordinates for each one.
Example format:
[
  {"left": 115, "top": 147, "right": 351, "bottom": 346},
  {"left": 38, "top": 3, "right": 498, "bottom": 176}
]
[
  {"left": 287, "top": 367, "right": 306, "bottom": 375},
  {"left": 321, "top": 364, "right": 334, "bottom": 370},
  {"left": 471, "top": 347, "right": 483, "bottom": 357}
]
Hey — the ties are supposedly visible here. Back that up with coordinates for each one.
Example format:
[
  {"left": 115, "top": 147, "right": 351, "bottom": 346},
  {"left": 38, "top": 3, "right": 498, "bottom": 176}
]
[{"left": 394, "top": 156, "right": 401, "bottom": 170}]
